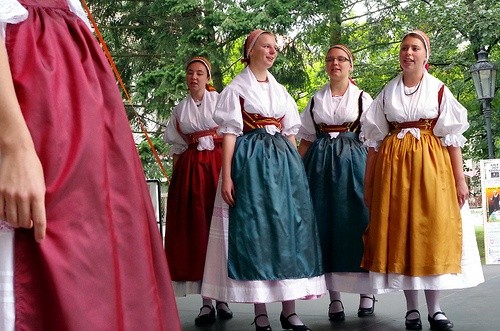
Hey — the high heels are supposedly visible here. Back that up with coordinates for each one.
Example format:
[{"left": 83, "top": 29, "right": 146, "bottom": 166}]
[{"left": 280, "top": 313, "right": 310, "bottom": 331}]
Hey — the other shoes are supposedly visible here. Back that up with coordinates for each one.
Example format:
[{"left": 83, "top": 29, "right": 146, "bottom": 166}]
[
  {"left": 428, "top": 311, "right": 453, "bottom": 330},
  {"left": 358, "top": 295, "right": 376, "bottom": 317},
  {"left": 217, "top": 302, "right": 233, "bottom": 327},
  {"left": 196, "top": 305, "right": 216, "bottom": 327},
  {"left": 254, "top": 314, "right": 272, "bottom": 331},
  {"left": 328, "top": 300, "right": 346, "bottom": 322},
  {"left": 405, "top": 310, "right": 422, "bottom": 331}
]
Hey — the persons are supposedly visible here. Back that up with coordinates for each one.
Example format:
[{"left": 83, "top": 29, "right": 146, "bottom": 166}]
[
  {"left": 357, "top": 31, "right": 484, "bottom": 331},
  {"left": 164, "top": 58, "right": 233, "bottom": 326},
  {"left": 296, "top": 44, "right": 378, "bottom": 322},
  {"left": 202, "top": 26, "right": 327, "bottom": 331},
  {"left": 0, "top": 0, "right": 179, "bottom": 331}
]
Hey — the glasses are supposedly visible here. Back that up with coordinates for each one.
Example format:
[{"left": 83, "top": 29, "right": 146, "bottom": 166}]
[{"left": 325, "top": 56, "right": 350, "bottom": 63}]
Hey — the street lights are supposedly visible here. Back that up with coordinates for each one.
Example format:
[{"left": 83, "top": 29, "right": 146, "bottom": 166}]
[{"left": 470, "top": 50, "right": 498, "bottom": 159}]
